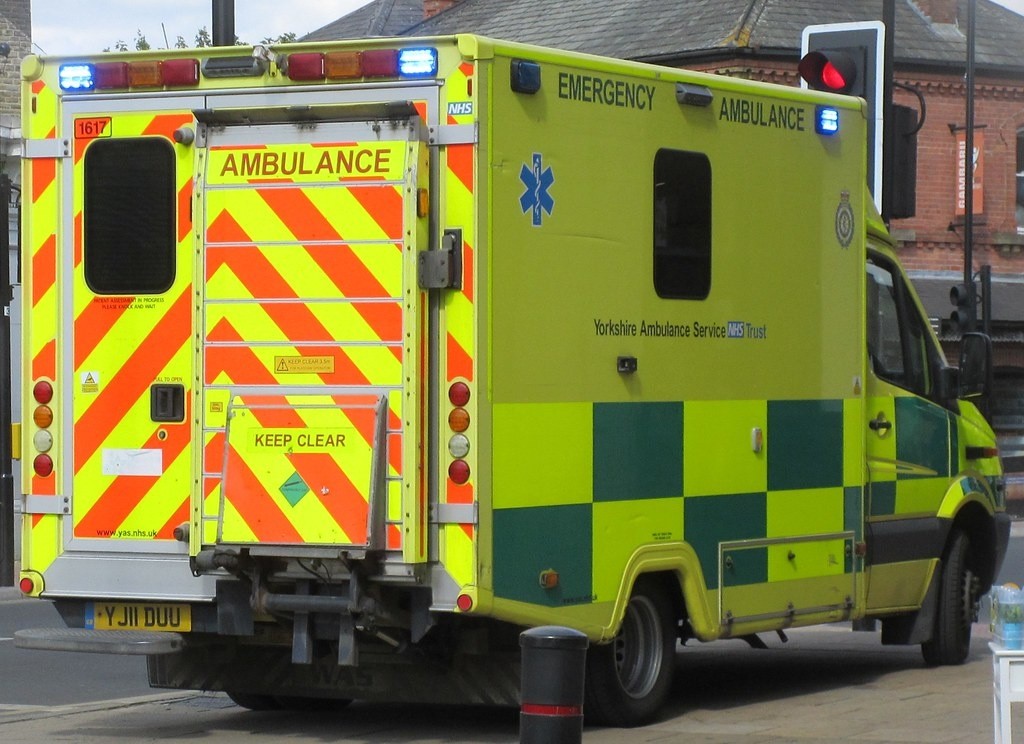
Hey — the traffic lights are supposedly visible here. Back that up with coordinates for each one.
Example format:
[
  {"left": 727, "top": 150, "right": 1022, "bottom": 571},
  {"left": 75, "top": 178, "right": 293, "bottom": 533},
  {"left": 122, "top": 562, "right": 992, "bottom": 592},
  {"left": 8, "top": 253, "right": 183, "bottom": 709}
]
[
  {"left": 798, "top": 19, "right": 884, "bottom": 227},
  {"left": 948, "top": 276, "right": 978, "bottom": 335}
]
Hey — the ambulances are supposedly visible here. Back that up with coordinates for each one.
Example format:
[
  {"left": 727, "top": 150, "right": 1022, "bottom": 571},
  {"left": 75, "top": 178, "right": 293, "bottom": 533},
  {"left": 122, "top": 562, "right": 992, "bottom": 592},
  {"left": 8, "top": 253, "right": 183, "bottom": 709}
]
[{"left": 15, "top": 33, "right": 1013, "bottom": 730}]
